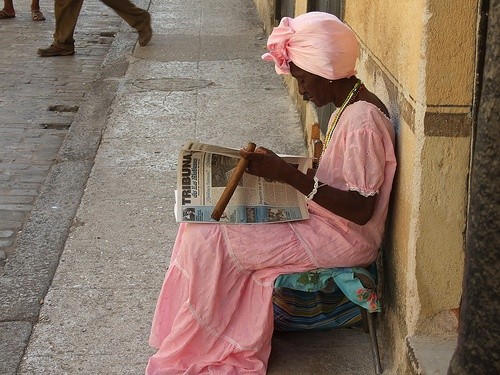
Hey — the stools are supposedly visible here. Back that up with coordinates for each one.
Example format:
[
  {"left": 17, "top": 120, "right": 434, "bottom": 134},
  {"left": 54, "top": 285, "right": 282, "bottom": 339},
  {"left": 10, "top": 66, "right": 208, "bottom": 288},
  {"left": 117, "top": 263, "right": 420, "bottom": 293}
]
[{"left": 273, "top": 261, "right": 384, "bottom": 375}]
